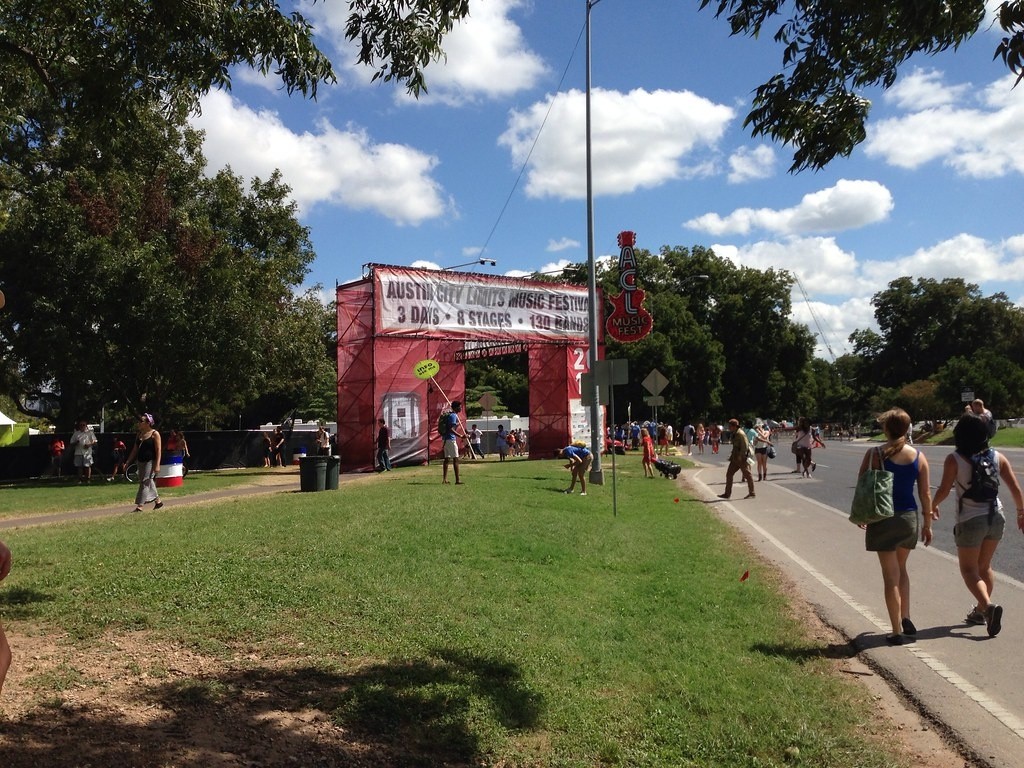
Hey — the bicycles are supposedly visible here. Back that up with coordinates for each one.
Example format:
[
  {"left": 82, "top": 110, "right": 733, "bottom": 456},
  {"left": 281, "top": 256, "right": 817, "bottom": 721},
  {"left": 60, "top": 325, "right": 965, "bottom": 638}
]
[{"left": 125, "top": 462, "right": 189, "bottom": 482}]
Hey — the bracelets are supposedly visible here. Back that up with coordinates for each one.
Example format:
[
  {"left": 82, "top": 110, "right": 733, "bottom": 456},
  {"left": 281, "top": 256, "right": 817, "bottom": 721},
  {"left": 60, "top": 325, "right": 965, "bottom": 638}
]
[
  {"left": 1016, "top": 508, "right": 1024, "bottom": 518},
  {"left": 922, "top": 511, "right": 933, "bottom": 516}
]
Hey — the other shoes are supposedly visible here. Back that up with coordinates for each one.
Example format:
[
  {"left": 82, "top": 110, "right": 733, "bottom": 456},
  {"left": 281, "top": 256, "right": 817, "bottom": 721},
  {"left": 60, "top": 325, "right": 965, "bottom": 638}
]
[
  {"left": 791, "top": 470, "right": 801, "bottom": 473},
  {"left": 562, "top": 488, "right": 574, "bottom": 494},
  {"left": 387, "top": 469, "right": 391, "bottom": 472},
  {"left": 885, "top": 632, "right": 903, "bottom": 645},
  {"left": 745, "top": 494, "right": 756, "bottom": 499},
  {"left": 454, "top": 482, "right": 465, "bottom": 485},
  {"left": 106, "top": 477, "right": 114, "bottom": 482},
  {"left": 812, "top": 463, "right": 816, "bottom": 471},
  {"left": 442, "top": 481, "right": 450, "bottom": 484},
  {"left": 580, "top": 491, "right": 587, "bottom": 495},
  {"left": 902, "top": 617, "right": 917, "bottom": 635},
  {"left": 717, "top": 494, "right": 731, "bottom": 499},
  {"left": 380, "top": 468, "right": 387, "bottom": 472},
  {"left": 757, "top": 473, "right": 767, "bottom": 481}
]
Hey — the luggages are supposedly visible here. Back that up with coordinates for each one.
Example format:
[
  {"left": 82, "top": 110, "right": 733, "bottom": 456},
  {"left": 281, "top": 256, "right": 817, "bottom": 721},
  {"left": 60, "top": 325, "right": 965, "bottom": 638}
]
[{"left": 652, "top": 455, "right": 681, "bottom": 479}]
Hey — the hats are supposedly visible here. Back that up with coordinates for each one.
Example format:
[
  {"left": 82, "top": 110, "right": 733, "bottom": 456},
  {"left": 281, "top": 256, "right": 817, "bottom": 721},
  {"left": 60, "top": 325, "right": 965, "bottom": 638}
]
[
  {"left": 145, "top": 412, "right": 155, "bottom": 427},
  {"left": 726, "top": 418, "right": 739, "bottom": 427}
]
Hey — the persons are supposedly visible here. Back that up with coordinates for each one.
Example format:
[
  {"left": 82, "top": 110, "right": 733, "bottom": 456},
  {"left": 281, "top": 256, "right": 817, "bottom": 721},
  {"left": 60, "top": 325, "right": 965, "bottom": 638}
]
[
  {"left": 123, "top": 413, "right": 164, "bottom": 513},
  {"left": 906, "top": 417, "right": 948, "bottom": 439},
  {"left": 373, "top": 418, "right": 391, "bottom": 471},
  {"left": 442, "top": 400, "right": 469, "bottom": 484},
  {"left": 0, "top": 542, "right": 12, "bottom": 694},
  {"left": 717, "top": 419, "right": 756, "bottom": 499},
  {"left": 262, "top": 426, "right": 339, "bottom": 468},
  {"left": 46, "top": 418, "right": 190, "bottom": 485},
  {"left": 965, "top": 399, "right": 992, "bottom": 448},
  {"left": 741, "top": 417, "right": 787, "bottom": 483},
  {"left": 606, "top": 420, "right": 723, "bottom": 456},
  {"left": 792, "top": 416, "right": 856, "bottom": 477},
  {"left": 857, "top": 408, "right": 934, "bottom": 645},
  {"left": 641, "top": 428, "right": 655, "bottom": 478},
  {"left": 931, "top": 411, "right": 1024, "bottom": 636},
  {"left": 462, "top": 424, "right": 527, "bottom": 460},
  {"left": 553, "top": 446, "right": 594, "bottom": 495}
]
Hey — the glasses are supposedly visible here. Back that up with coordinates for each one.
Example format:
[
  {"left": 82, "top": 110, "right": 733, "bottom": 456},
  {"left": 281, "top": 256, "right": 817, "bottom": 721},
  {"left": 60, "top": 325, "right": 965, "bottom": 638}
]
[
  {"left": 79, "top": 424, "right": 85, "bottom": 426},
  {"left": 138, "top": 419, "right": 146, "bottom": 423}
]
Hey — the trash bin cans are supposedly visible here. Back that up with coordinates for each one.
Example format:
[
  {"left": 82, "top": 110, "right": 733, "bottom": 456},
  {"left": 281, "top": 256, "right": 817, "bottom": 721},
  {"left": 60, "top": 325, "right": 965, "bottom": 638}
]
[
  {"left": 327, "top": 455, "right": 340, "bottom": 491},
  {"left": 299, "top": 456, "right": 328, "bottom": 492}
]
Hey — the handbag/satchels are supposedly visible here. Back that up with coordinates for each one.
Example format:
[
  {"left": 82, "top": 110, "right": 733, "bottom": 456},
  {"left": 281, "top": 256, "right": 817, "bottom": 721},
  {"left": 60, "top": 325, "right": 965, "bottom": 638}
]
[
  {"left": 849, "top": 468, "right": 896, "bottom": 525},
  {"left": 792, "top": 441, "right": 798, "bottom": 455},
  {"left": 766, "top": 445, "right": 776, "bottom": 459},
  {"left": 570, "top": 441, "right": 587, "bottom": 448}
]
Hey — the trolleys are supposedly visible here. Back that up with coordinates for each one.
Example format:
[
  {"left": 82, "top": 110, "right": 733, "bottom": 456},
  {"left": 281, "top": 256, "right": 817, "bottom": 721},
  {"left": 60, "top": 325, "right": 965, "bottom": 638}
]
[{"left": 650, "top": 458, "right": 682, "bottom": 479}]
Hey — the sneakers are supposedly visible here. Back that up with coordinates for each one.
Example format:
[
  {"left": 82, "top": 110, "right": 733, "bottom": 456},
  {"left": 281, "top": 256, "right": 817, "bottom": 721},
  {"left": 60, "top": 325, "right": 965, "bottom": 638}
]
[
  {"left": 967, "top": 606, "right": 987, "bottom": 625},
  {"left": 153, "top": 501, "right": 163, "bottom": 510},
  {"left": 131, "top": 507, "right": 143, "bottom": 513},
  {"left": 983, "top": 604, "right": 1003, "bottom": 636}
]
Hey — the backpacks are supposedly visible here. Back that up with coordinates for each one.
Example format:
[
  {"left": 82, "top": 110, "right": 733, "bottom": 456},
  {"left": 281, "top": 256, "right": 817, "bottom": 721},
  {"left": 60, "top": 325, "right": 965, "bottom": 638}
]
[
  {"left": 438, "top": 412, "right": 453, "bottom": 439},
  {"left": 955, "top": 452, "right": 1002, "bottom": 507}
]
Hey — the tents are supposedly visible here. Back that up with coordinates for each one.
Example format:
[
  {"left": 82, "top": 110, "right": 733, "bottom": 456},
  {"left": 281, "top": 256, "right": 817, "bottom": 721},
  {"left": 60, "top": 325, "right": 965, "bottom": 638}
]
[{"left": 0, "top": 411, "right": 17, "bottom": 446}]
